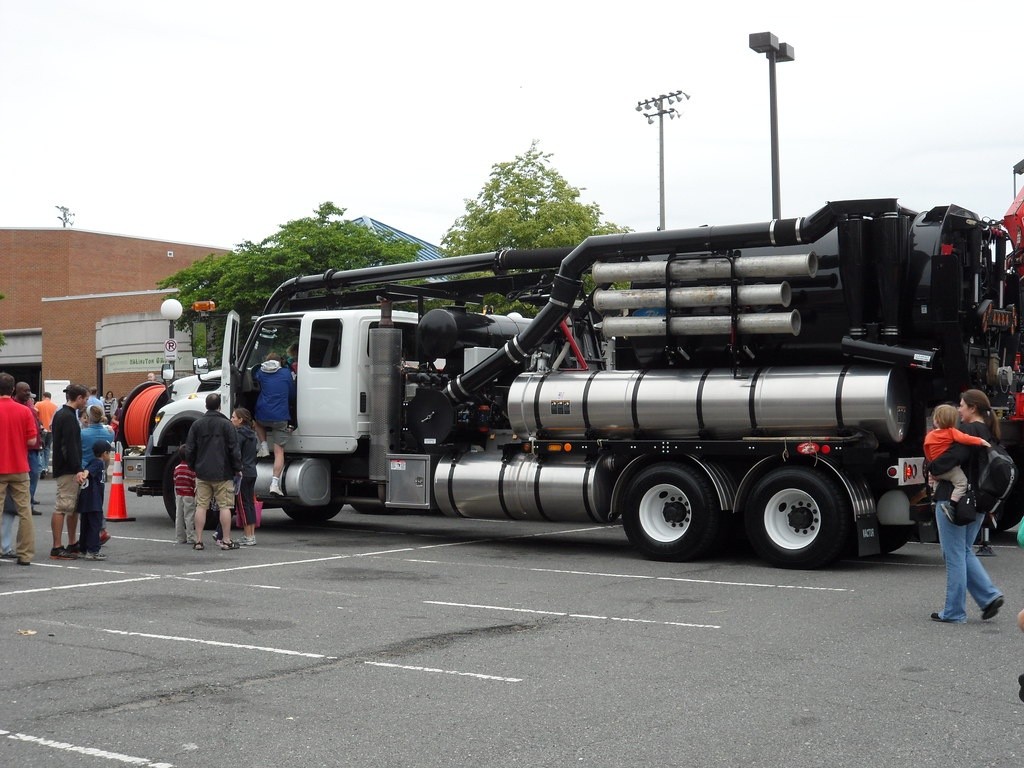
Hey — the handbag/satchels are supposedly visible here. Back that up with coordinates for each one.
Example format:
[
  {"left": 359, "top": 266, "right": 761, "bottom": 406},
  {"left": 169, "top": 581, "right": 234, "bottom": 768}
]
[
  {"left": 236, "top": 493, "right": 263, "bottom": 528},
  {"left": 951, "top": 495, "right": 976, "bottom": 526}
]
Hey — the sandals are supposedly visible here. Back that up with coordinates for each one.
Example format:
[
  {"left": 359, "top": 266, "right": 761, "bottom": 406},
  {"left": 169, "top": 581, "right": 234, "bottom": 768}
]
[
  {"left": 222, "top": 540, "right": 240, "bottom": 550},
  {"left": 193, "top": 542, "right": 204, "bottom": 550}
]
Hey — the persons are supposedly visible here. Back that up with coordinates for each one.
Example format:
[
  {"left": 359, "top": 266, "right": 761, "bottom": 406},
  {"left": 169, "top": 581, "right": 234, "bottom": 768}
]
[
  {"left": 104, "top": 391, "right": 117, "bottom": 423},
  {"left": 230, "top": 408, "right": 256, "bottom": 545},
  {"left": 36, "top": 393, "right": 58, "bottom": 479},
  {"left": 185, "top": 392, "right": 239, "bottom": 550},
  {"left": 80, "top": 439, "right": 116, "bottom": 560},
  {"left": 89, "top": 388, "right": 103, "bottom": 407},
  {"left": 112, "top": 396, "right": 126, "bottom": 427},
  {"left": 923, "top": 404, "right": 993, "bottom": 522},
  {"left": 172, "top": 444, "right": 197, "bottom": 544},
  {"left": 51, "top": 384, "right": 90, "bottom": 561},
  {"left": 0, "top": 373, "right": 35, "bottom": 566},
  {"left": 79, "top": 404, "right": 115, "bottom": 544},
  {"left": 0, "top": 382, "right": 48, "bottom": 557},
  {"left": 253, "top": 352, "right": 296, "bottom": 495},
  {"left": 927, "top": 389, "right": 1003, "bottom": 623}
]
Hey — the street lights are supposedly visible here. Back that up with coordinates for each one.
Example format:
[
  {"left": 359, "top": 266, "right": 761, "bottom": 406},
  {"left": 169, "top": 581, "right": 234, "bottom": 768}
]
[
  {"left": 1011, "top": 160, "right": 1024, "bottom": 201},
  {"left": 634, "top": 88, "right": 690, "bottom": 230},
  {"left": 747, "top": 30, "right": 796, "bottom": 219},
  {"left": 159, "top": 299, "right": 184, "bottom": 384}
]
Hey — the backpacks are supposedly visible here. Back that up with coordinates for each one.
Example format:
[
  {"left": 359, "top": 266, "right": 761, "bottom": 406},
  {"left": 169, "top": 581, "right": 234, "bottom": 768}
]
[{"left": 968, "top": 425, "right": 1014, "bottom": 514}]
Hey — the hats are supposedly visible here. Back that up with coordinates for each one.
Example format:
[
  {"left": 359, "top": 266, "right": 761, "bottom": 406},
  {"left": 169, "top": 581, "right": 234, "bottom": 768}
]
[
  {"left": 87, "top": 406, "right": 103, "bottom": 422},
  {"left": 92, "top": 440, "right": 115, "bottom": 451}
]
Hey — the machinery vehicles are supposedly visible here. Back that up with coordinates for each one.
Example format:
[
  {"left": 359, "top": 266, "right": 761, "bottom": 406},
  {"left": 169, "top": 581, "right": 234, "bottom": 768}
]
[{"left": 119, "top": 186, "right": 1024, "bottom": 572}]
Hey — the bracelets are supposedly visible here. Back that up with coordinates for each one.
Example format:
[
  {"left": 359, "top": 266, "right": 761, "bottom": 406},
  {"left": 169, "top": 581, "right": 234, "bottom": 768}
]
[{"left": 41, "top": 428, "right": 44, "bottom": 431}]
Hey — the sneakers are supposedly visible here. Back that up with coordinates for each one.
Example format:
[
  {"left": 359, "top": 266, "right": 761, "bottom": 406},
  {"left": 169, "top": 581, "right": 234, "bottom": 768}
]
[
  {"left": 257, "top": 448, "right": 270, "bottom": 457},
  {"left": 85, "top": 552, "right": 107, "bottom": 560},
  {"left": 252, "top": 536, "right": 256, "bottom": 544},
  {"left": 67, "top": 543, "right": 80, "bottom": 553},
  {"left": 79, "top": 551, "right": 85, "bottom": 557},
  {"left": 236, "top": 535, "right": 253, "bottom": 546},
  {"left": 942, "top": 501, "right": 955, "bottom": 521},
  {"left": 50, "top": 546, "right": 75, "bottom": 560},
  {"left": 268, "top": 487, "right": 284, "bottom": 497}
]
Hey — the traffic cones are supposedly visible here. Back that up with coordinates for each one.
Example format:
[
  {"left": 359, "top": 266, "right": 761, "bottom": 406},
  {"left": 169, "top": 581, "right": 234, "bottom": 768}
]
[{"left": 105, "top": 451, "right": 137, "bottom": 523}]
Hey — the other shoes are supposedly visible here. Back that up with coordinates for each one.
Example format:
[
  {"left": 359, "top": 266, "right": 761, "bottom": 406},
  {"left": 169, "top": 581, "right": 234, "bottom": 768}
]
[
  {"left": 982, "top": 597, "right": 1004, "bottom": 620},
  {"left": 32, "top": 509, "right": 42, "bottom": 515},
  {"left": 16, "top": 558, "right": 29, "bottom": 566},
  {"left": 100, "top": 532, "right": 111, "bottom": 544},
  {"left": 216, "top": 540, "right": 222, "bottom": 546},
  {"left": 931, "top": 613, "right": 954, "bottom": 623},
  {"left": 212, "top": 532, "right": 219, "bottom": 541},
  {"left": 33, "top": 501, "right": 40, "bottom": 504},
  {"left": 1, "top": 550, "right": 17, "bottom": 560}
]
